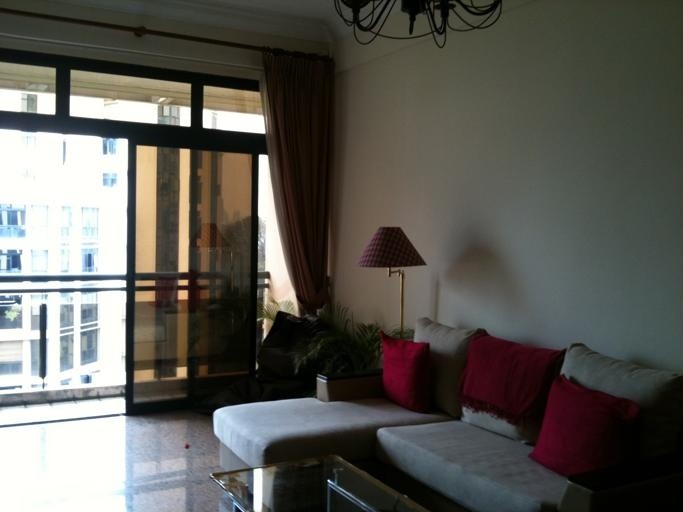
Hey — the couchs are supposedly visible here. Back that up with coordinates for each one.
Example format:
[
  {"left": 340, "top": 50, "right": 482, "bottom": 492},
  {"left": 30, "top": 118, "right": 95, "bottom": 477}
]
[{"left": 209, "top": 316, "right": 681, "bottom": 512}]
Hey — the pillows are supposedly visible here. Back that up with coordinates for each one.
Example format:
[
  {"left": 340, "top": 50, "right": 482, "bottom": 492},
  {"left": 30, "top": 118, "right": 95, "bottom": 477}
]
[
  {"left": 378, "top": 328, "right": 431, "bottom": 414},
  {"left": 526, "top": 373, "right": 642, "bottom": 488}
]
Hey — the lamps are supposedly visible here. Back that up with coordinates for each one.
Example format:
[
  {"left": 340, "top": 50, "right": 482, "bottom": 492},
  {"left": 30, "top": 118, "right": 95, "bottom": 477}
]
[
  {"left": 355, "top": 224, "right": 427, "bottom": 338},
  {"left": 332, "top": 0, "right": 505, "bottom": 49}
]
